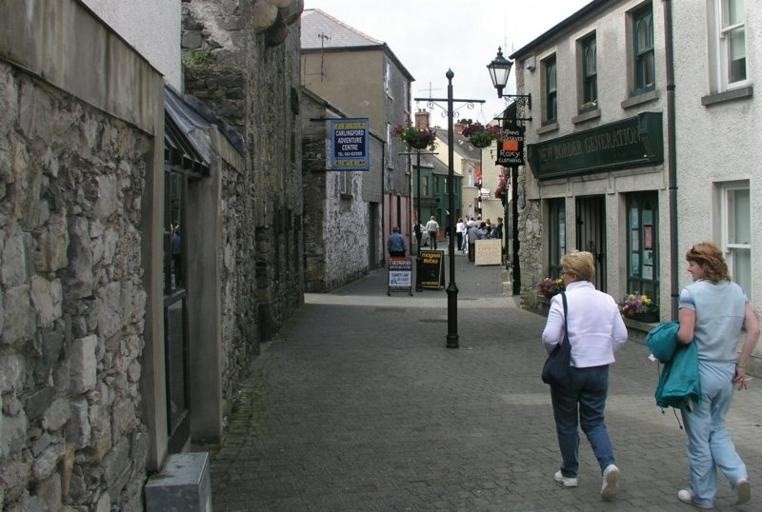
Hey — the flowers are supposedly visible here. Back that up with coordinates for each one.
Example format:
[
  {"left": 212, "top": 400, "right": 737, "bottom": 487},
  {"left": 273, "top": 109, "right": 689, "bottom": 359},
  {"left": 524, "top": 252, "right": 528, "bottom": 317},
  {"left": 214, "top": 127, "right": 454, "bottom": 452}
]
[
  {"left": 538, "top": 276, "right": 565, "bottom": 296},
  {"left": 392, "top": 121, "right": 441, "bottom": 153},
  {"left": 616, "top": 291, "right": 656, "bottom": 321},
  {"left": 460, "top": 121, "right": 503, "bottom": 145}
]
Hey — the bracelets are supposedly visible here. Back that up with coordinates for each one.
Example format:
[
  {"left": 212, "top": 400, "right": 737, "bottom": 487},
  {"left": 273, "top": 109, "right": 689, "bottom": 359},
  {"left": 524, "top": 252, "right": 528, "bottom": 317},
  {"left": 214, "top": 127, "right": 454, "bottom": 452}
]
[{"left": 734, "top": 361, "right": 748, "bottom": 370}]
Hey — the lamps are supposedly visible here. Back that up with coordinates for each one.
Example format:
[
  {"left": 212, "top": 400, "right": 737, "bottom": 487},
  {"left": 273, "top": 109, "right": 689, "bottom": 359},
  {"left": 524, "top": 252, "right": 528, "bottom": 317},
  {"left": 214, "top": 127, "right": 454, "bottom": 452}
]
[{"left": 486, "top": 45, "right": 531, "bottom": 111}]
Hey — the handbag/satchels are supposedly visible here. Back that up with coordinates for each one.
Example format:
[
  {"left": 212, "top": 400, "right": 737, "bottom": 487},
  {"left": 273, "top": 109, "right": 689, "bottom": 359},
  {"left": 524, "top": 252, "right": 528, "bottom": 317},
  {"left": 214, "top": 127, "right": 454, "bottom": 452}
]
[{"left": 542, "top": 336, "right": 571, "bottom": 388}]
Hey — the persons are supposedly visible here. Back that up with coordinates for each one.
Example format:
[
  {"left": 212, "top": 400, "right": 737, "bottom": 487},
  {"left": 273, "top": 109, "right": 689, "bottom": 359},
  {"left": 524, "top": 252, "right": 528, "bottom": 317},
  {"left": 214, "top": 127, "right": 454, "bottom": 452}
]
[
  {"left": 676, "top": 241, "right": 760, "bottom": 509},
  {"left": 541, "top": 249, "right": 629, "bottom": 503},
  {"left": 386, "top": 212, "right": 503, "bottom": 258}
]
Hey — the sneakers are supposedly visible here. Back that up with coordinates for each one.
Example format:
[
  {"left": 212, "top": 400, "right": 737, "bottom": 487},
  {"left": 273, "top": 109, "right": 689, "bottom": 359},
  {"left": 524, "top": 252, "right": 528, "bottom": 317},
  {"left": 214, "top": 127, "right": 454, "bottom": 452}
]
[
  {"left": 732, "top": 479, "right": 751, "bottom": 506},
  {"left": 678, "top": 489, "right": 713, "bottom": 510},
  {"left": 600, "top": 465, "right": 619, "bottom": 498},
  {"left": 556, "top": 471, "right": 577, "bottom": 486}
]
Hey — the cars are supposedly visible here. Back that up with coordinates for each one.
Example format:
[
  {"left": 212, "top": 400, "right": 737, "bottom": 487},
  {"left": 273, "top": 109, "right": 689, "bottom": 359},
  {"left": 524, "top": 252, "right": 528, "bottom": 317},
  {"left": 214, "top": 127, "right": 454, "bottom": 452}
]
[{"left": 412, "top": 223, "right": 430, "bottom": 246}]
